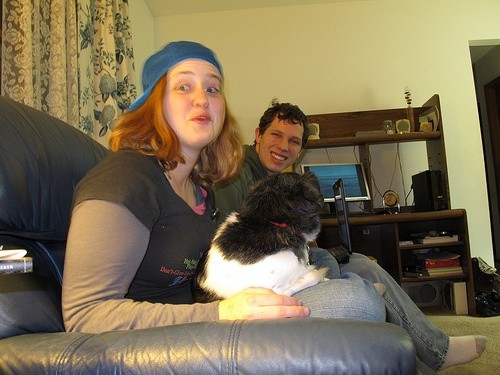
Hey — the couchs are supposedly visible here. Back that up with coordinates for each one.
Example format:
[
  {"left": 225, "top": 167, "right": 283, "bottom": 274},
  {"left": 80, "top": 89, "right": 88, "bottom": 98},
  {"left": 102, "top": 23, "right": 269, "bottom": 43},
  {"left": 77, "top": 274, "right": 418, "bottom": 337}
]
[{"left": 0, "top": 91, "right": 459, "bottom": 375}]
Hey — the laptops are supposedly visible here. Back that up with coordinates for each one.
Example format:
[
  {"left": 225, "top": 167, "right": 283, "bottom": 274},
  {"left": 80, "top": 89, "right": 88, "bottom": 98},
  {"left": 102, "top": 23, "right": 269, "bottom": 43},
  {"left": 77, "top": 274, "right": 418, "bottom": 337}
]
[{"left": 327, "top": 178, "right": 354, "bottom": 264}]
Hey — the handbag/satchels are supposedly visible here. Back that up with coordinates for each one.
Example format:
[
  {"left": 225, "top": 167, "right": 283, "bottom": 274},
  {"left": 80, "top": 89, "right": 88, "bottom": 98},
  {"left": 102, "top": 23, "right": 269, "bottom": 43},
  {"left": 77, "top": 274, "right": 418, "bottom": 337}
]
[{"left": 470, "top": 257, "right": 500, "bottom": 318}]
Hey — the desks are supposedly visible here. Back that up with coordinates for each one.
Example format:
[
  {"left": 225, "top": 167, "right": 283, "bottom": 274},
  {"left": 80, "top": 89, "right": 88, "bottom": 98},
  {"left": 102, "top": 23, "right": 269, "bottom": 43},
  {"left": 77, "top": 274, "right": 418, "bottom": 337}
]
[{"left": 272, "top": 94, "right": 469, "bottom": 316}]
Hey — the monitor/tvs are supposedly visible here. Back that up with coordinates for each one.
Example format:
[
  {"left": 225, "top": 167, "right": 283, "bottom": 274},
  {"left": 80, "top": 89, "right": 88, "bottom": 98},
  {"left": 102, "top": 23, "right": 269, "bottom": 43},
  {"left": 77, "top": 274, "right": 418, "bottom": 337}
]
[{"left": 300, "top": 162, "right": 372, "bottom": 214}]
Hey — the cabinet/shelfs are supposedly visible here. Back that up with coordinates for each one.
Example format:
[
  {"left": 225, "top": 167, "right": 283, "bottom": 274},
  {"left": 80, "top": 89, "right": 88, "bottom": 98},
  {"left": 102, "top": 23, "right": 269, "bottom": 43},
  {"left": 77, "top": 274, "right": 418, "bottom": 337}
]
[{"left": 394, "top": 211, "right": 472, "bottom": 282}]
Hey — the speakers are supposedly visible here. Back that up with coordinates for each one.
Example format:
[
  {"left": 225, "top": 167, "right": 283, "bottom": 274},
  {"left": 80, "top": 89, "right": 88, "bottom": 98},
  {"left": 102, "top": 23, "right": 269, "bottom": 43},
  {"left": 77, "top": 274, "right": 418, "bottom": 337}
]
[
  {"left": 382, "top": 190, "right": 400, "bottom": 212},
  {"left": 412, "top": 170, "right": 448, "bottom": 212}
]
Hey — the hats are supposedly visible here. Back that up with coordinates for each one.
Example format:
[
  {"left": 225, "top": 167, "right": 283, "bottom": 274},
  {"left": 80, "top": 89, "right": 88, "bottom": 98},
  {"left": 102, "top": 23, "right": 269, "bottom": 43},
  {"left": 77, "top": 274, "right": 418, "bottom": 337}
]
[{"left": 124, "top": 41, "right": 218, "bottom": 112}]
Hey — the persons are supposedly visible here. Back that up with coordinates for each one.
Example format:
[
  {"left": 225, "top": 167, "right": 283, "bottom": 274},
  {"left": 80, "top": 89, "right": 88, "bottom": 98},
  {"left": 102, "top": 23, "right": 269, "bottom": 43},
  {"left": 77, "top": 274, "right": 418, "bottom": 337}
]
[
  {"left": 202, "top": 103, "right": 489, "bottom": 370},
  {"left": 60, "top": 41, "right": 387, "bottom": 332}
]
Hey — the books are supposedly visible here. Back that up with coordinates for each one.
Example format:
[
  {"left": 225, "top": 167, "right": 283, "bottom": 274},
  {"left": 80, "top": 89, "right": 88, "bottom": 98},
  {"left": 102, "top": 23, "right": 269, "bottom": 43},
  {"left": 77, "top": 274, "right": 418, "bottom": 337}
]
[{"left": 415, "top": 250, "right": 463, "bottom": 276}]
